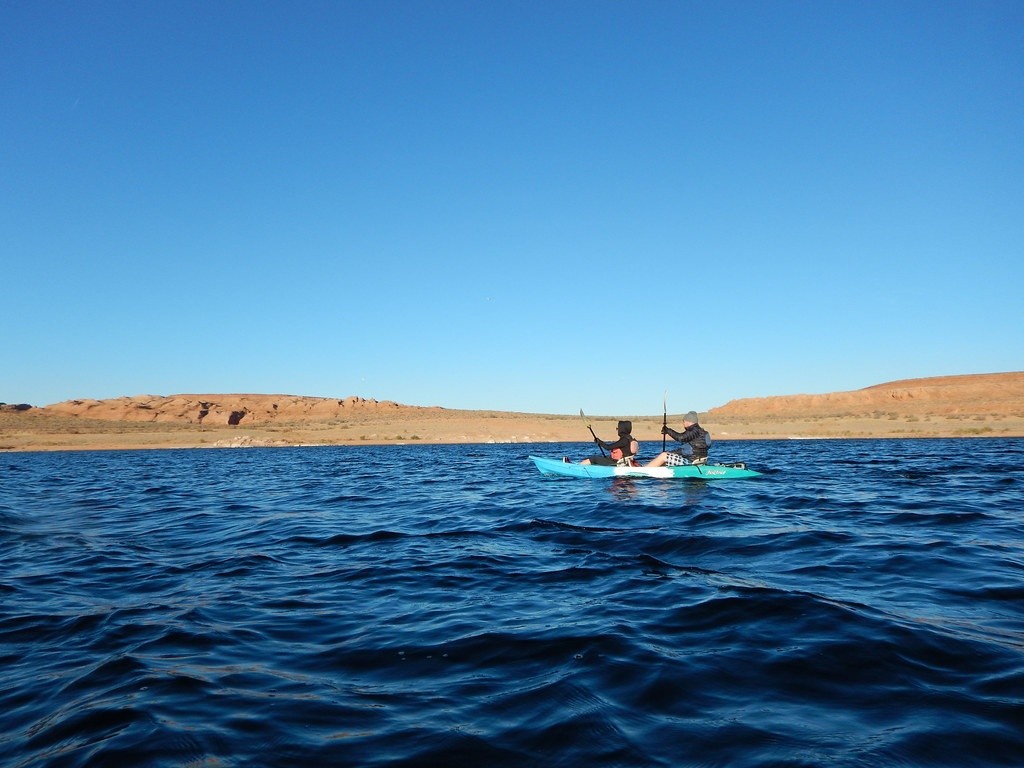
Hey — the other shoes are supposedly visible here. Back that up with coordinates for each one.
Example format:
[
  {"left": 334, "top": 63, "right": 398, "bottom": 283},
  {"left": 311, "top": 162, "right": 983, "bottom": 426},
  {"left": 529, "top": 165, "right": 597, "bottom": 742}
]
[
  {"left": 628, "top": 459, "right": 642, "bottom": 467},
  {"left": 563, "top": 457, "right": 571, "bottom": 463}
]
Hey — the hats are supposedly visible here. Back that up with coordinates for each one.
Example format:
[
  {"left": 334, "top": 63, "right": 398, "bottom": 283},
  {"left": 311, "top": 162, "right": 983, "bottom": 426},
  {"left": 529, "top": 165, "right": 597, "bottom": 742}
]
[{"left": 683, "top": 411, "right": 698, "bottom": 422}]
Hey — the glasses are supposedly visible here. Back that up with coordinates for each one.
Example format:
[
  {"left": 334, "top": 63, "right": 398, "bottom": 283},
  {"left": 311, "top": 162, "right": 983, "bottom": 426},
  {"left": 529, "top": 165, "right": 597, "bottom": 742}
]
[{"left": 616, "top": 428, "right": 619, "bottom": 430}]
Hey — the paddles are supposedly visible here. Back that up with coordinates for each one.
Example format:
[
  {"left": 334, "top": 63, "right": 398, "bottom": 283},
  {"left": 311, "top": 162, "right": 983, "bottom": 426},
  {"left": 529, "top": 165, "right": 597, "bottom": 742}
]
[
  {"left": 662, "top": 389, "right": 669, "bottom": 452},
  {"left": 580, "top": 408, "right": 607, "bottom": 458}
]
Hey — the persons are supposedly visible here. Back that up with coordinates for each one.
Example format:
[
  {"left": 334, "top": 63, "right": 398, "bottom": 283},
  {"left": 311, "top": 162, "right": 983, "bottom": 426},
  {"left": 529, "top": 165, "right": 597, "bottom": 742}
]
[
  {"left": 563, "top": 420, "right": 639, "bottom": 466},
  {"left": 628, "top": 411, "right": 711, "bottom": 467}
]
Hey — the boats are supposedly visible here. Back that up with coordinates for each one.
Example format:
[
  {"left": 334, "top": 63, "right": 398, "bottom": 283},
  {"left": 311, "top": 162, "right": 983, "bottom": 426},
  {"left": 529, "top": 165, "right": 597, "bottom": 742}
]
[{"left": 529, "top": 454, "right": 767, "bottom": 479}]
